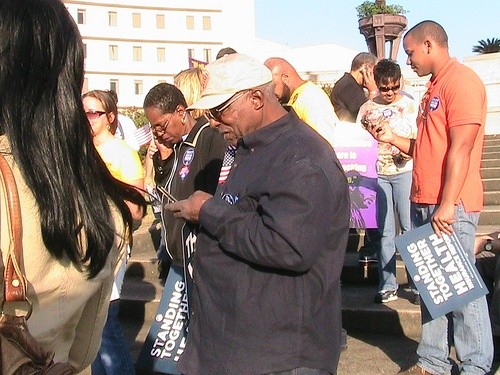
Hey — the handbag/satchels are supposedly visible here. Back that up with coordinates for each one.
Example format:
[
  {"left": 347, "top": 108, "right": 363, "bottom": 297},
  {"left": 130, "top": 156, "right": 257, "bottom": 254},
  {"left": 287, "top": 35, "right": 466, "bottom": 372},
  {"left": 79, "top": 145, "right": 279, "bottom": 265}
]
[{"left": 0, "top": 316, "right": 75, "bottom": 374}]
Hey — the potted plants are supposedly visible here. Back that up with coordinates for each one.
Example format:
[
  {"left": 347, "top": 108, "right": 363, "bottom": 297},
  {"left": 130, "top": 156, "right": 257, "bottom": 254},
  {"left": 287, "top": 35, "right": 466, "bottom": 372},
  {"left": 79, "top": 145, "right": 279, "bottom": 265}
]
[{"left": 355, "top": 0, "right": 408, "bottom": 62}]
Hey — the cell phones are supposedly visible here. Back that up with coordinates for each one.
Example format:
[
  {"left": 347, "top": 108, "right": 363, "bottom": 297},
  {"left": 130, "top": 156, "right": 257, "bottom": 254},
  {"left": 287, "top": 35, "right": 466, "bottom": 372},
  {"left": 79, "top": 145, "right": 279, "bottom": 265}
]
[
  {"left": 360, "top": 66, "right": 371, "bottom": 77},
  {"left": 362, "top": 119, "right": 383, "bottom": 133},
  {"left": 155, "top": 184, "right": 178, "bottom": 205}
]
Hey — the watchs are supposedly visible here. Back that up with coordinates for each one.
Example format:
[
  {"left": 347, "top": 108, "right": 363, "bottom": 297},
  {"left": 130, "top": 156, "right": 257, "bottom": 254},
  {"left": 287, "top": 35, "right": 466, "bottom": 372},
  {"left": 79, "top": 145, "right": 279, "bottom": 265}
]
[{"left": 484, "top": 239, "right": 493, "bottom": 251}]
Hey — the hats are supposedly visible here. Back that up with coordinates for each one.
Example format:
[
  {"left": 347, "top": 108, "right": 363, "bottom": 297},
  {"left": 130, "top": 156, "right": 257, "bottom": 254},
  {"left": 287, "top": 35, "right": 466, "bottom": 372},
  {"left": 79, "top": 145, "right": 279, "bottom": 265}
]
[{"left": 187, "top": 53, "right": 272, "bottom": 110}]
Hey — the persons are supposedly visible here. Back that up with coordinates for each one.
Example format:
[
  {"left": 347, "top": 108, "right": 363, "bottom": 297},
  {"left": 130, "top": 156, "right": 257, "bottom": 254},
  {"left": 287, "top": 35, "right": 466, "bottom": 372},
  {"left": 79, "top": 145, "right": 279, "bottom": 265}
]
[
  {"left": 145, "top": 69, "right": 207, "bottom": 189},
  {"left": 81, "top": 90, "right": 142, "bottom": 375},
  {"left": 474, "top": 230, "right": 500, "bottom": 256},
  {"left": 263, "top": 57, "right": 342, "bottom": 157},
  {"left": 145, "top": 84, "right": 231, "bottom": 286},
  {"left": 356, "top": 59, "right": 419, "bottom": 303},
  {"left": 165, "top": 54, "right": 349, "bottom": 375},
  {"left": 0, "top": 0, "right": 126, "bottom": 375},
  {"left": 361, "top": 20, "right": 494, "bottom": 375},
  {"left": 105, "top": 92, "right": 140, "bottom": 153},
  {"left": 328, "top": 52, "right": 380, "bottom": 284}
]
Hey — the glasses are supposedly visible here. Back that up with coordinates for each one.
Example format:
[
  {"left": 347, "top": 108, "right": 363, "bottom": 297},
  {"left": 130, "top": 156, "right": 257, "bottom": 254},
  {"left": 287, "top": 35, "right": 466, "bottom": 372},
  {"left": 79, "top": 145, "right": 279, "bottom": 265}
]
[
  {"left": 86, "top": 110, "right": 110, "bottom": 118},
  {"left": 152, "top": 111, "right": 178, "bottom": 134},
  {"left": 377, "top": 84, "right": 401, "bottom": 91},
  {"left": 204, "top": 92, "right": 250, "bottom": 121}
]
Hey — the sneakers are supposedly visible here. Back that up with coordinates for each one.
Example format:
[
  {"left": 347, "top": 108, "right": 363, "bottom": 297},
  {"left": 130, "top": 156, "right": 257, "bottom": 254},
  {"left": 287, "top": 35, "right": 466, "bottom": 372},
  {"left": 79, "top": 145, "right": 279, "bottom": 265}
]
[
  {"left": 375, "top": 289, "right": 399, "bottom": 303},
  {"left": 398, "top": 365, "right": 437, "bottom": 375}
]
